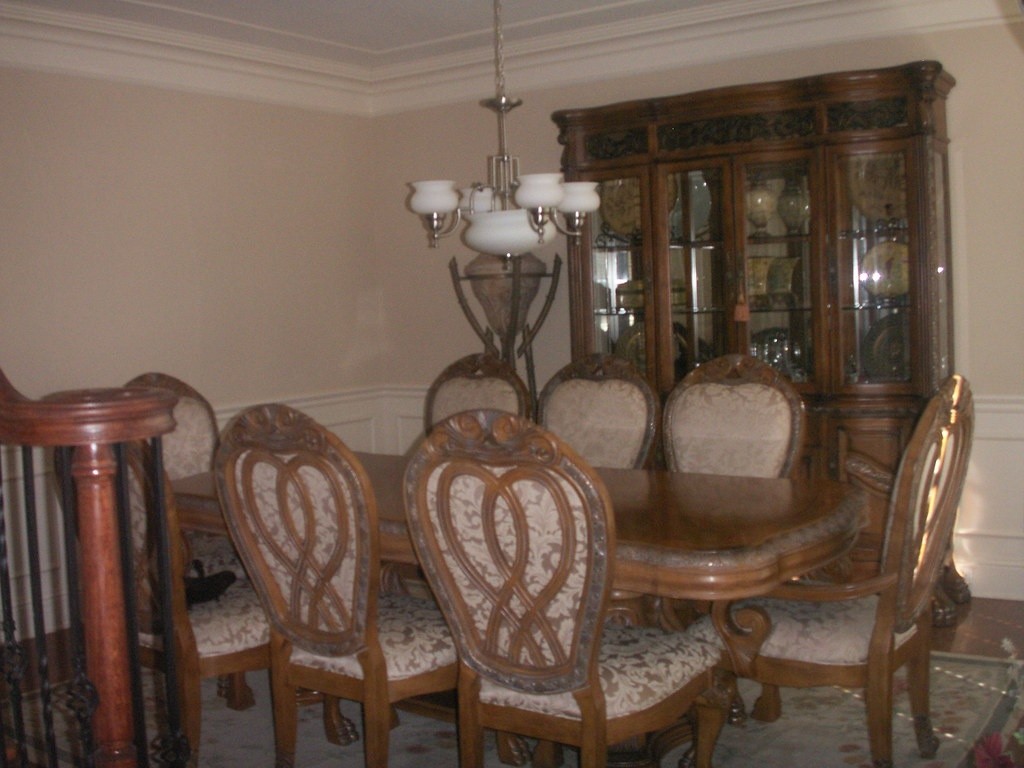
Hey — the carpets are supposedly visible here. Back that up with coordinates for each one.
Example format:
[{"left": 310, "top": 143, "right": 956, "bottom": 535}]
[{"left": 0, "top": 652, "right": 1024, "bottom": 768}]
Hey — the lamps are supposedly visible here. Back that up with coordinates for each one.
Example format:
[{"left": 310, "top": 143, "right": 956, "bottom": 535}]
[{"left": 410, "top": 0, "right": 601, "bottom": 259}]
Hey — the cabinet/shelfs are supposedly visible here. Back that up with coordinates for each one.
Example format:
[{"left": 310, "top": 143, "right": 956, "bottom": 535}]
[{"left": 550, "top": 60, "right": 972, "bottom": 629}]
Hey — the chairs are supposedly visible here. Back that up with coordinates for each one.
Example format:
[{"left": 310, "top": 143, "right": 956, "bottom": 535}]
[
  {"left": 711, "top": 372, "right": 975, "bottom": 767},
  {"left": 210, "top": 403, "right": 459, "bottom": 768},
  {"left": 536, "top": 353, "right": 657, "bottom": 633},
  {"left": 375, "top": 352, "right": 530, "bottom": 595},
  {"left": 400, "top": 409, "right": 722, "bottom": 767},
  {"left": 661, "top": 353, "right": 805, "bottom": 478},
  {"left": 53, "top": 371, "right": 360, "bottom": 767}
]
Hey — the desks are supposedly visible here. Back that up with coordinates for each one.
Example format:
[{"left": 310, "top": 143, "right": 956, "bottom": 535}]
[{"left": 172, "top": 450, "right": 868, "bottom": 768}]
